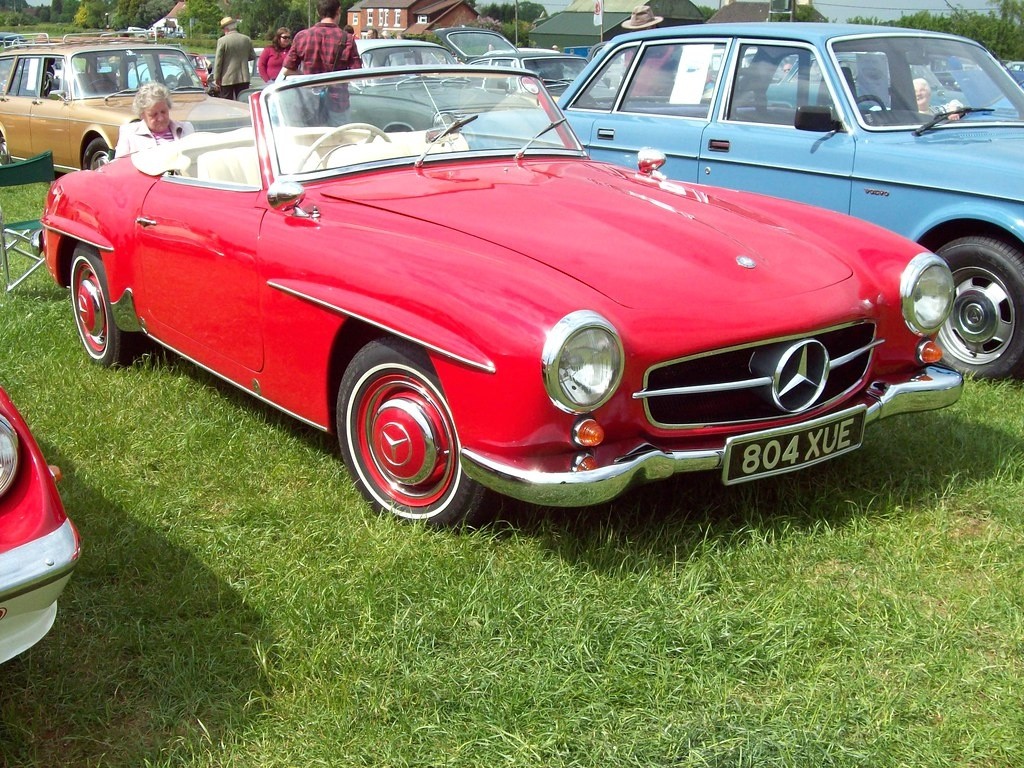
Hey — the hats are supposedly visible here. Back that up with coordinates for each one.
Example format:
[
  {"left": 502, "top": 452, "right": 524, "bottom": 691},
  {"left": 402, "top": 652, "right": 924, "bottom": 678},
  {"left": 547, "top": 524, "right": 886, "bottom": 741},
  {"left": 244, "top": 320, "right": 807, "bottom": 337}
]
[
  {"left": 219, "top": 17, "right": 237, "bottom": 29},
  {"left": 621, "top": 6, "right": 664, "bottom": 29}
]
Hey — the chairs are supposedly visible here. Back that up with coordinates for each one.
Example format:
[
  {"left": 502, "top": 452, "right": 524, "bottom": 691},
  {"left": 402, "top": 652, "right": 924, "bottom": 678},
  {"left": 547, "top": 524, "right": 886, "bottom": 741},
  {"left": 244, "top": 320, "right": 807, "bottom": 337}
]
[
  {"left": 0, "top": 149, "right": 54, "bottom": 292},
  {"left": 819, "top": 67, "right": 859, "bottom": 109},
  {"left": 731, "top": 63, "right": 774, "bottom": 122}
]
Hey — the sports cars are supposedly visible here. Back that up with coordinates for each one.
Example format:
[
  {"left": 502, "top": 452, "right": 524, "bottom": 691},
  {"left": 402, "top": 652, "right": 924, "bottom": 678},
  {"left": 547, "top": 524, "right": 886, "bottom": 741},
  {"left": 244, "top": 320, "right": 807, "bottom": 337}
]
[{"left": 31, "top": 59, "right": 965, "bottom": 536}]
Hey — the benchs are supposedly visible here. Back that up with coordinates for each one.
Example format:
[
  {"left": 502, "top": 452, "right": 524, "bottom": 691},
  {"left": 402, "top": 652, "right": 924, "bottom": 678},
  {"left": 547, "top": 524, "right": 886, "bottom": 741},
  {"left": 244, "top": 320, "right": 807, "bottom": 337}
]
[
  {"left": 74, "top": 72, "right": 117, "bottom": 95},
  {"left": 184, "top": 129, "right": 369, "bottom": 187},
  {"left": 626, "top": 106, "right": 798, "bottom": 126}
]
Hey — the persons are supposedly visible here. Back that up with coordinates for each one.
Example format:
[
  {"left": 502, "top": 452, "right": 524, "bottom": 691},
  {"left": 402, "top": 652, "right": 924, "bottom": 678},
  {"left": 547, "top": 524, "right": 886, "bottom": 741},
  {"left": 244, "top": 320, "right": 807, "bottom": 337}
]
[
  {"left": 946, "top": 54, "right": 962, "bottom": 70},
  {"left": 114, "top": 83, "right": 195, "bottom": 159},
  {"left": 381, "top": 31, "right": 393, "bottom": 39},
  {"left": 783, "top": 64, "right": 792, "bottom": 74},
  {"left": 275, "top": 0, "right": 362, "bottom": 127},
  {"left": 258, "top": 27, "right": 293, "bottom": 88},
  {"left": 913, "top": 78, "right": 964, "bottom": 120},
  {"left": 621, "top": 6, "right": 683, "bottom": 96},
  {"left": 366, "top": 29, "right": 378, "bottom": 39},
  {"left": 214, "top": 17, "right": 257, "bottom": 100},
  {"left": 397, "top": 33, "right": 406, "bottom": 39}
]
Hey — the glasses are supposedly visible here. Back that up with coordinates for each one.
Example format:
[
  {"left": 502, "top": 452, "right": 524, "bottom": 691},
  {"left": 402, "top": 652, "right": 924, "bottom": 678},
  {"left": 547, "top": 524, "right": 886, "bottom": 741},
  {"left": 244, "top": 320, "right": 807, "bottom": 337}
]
[{"left": 280, "top": 36, "right": 291, "bottom": 39}]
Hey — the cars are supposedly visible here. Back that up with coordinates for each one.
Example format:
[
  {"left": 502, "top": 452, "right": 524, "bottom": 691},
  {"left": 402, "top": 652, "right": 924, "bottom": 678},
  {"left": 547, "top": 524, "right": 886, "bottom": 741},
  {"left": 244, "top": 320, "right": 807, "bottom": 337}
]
[
  {"left": 458, "top": 21, "right": 1024, "bottom": 385},
  {"left": 0, "top": 27, "right": 1023, "bottom": 175}
]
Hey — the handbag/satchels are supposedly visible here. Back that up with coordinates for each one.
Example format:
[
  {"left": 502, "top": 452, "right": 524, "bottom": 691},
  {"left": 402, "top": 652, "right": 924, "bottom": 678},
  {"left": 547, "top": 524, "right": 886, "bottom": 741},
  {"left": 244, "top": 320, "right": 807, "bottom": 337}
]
[{"left": 280, "top": 86, "right": 330, "bottom": 125}]
